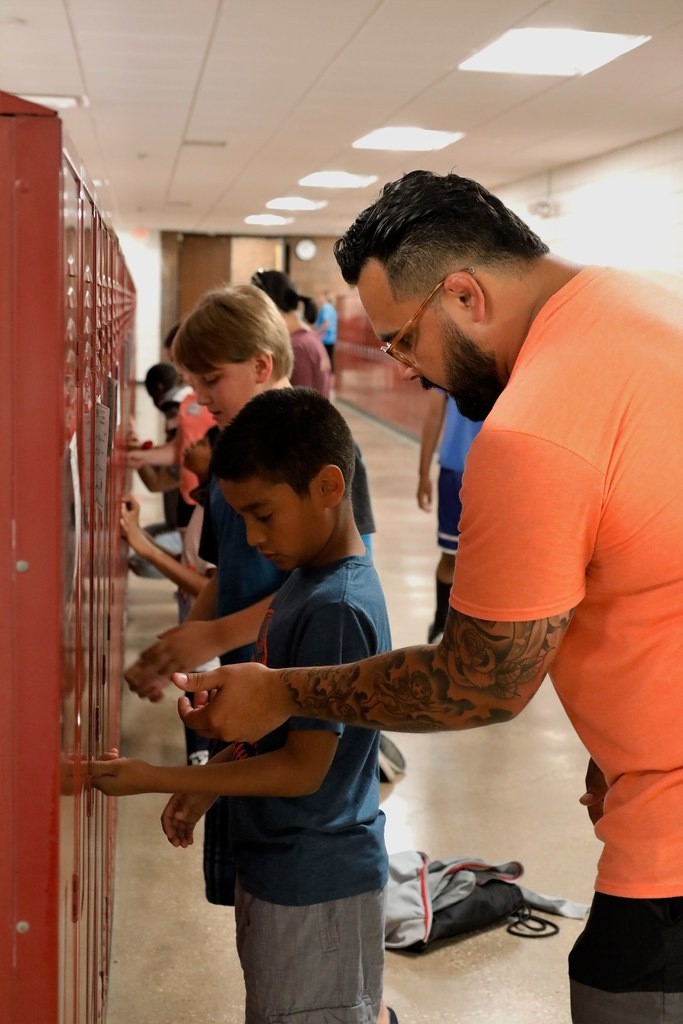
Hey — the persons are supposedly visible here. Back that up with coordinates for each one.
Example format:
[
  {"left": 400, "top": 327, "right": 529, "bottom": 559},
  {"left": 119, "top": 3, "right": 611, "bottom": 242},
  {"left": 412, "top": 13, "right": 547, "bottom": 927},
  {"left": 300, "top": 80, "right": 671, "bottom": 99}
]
[
  {"left": 92, "top": 388, "right": 391, "bottom": 1023},
  {"left": 313, "top": 287, "right": 337, "bottom": 402},
  {"left": 120, "top": 268, "right": 377, "bottom": 905},
  {"left": 172, "top": 170, "right": 683, "bottom": 1024},
  {"left": 416, "top": 388, "right": 485, "bottom": 644}
]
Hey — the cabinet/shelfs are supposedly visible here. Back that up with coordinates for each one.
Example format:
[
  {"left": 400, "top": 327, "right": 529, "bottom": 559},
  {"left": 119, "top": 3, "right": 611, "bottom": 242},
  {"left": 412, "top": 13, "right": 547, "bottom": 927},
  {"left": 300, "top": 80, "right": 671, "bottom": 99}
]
[
  {"left": 0, "top": 87, "right": 138, "bottom": 1024},
  {"left": 336, "top": 292, "right": 431, "bottom": 447}
]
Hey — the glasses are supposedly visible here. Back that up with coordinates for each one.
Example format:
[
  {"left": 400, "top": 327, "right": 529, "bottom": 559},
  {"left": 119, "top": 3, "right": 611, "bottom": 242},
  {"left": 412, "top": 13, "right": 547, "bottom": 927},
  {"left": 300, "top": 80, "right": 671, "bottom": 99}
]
[
  {"left": 251, "top": 271, "right": 267, "bottom": 291},
  {"left": 380, "top": 266, "right": 476, "bottom": 367}
]
